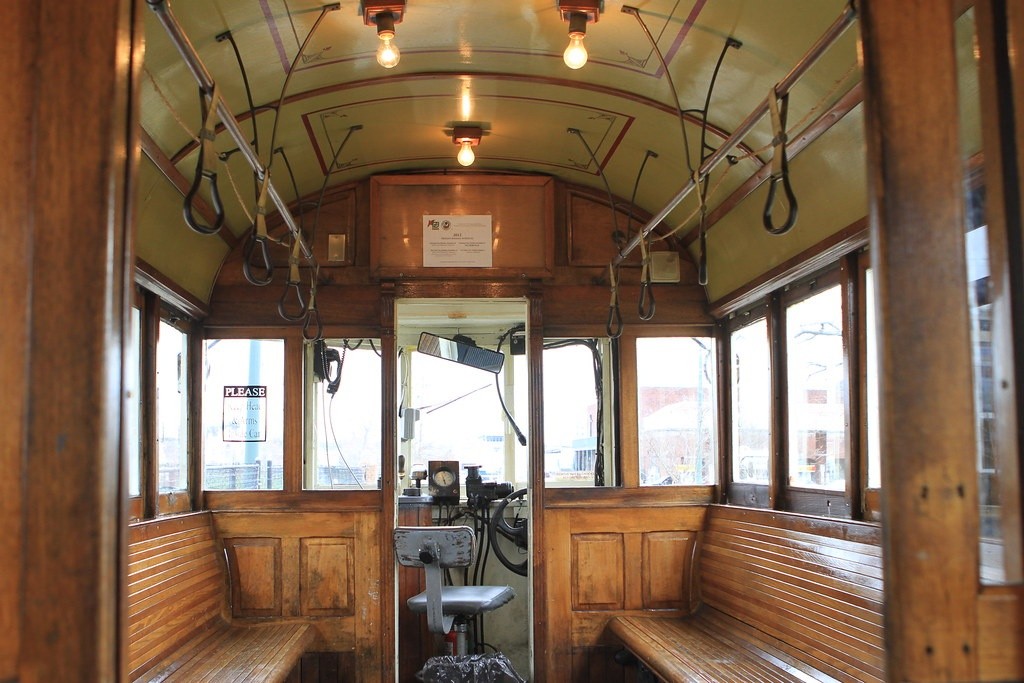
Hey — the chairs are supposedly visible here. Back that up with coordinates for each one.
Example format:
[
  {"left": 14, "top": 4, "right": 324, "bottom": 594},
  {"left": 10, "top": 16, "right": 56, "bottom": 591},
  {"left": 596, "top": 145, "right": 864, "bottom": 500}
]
[{"left": 393, "top": 524, "right": 518, "bottom": 683}]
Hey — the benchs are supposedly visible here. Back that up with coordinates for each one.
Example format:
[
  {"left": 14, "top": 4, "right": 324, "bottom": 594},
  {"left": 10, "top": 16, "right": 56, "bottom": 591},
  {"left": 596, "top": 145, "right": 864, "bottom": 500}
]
[
  {"left": 607, "top": 504, "right": 888, "bottom": 683},
  {"left": 127, "top": 510, "right": 318, "bottom": 683}
]
